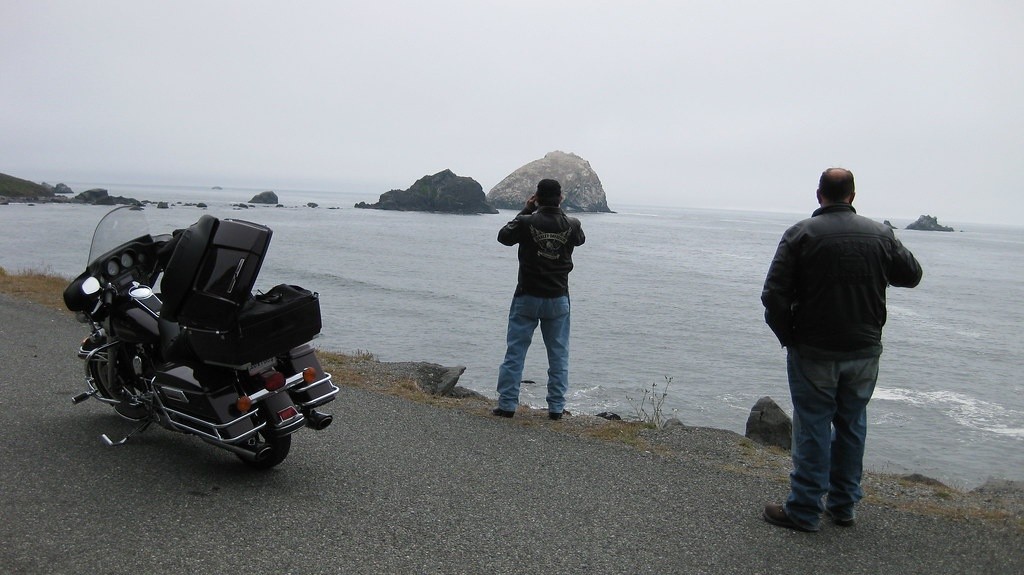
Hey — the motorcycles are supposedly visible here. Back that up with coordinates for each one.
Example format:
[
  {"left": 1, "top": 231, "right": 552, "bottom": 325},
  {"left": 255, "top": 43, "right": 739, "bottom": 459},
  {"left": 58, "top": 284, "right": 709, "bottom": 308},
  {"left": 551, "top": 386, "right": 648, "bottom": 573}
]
[{"left": 62, "top": 205, "right": 341, "bottom": 474}]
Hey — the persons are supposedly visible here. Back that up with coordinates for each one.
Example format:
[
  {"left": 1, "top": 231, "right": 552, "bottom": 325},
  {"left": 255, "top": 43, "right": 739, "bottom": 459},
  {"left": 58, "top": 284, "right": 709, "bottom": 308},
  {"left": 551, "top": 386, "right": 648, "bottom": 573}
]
[
  {"left": 761, "top": 167, "right": 923, "bottom": 533},
  {"left": 491, "top": 179, "right": 585, "bottom": 419}
]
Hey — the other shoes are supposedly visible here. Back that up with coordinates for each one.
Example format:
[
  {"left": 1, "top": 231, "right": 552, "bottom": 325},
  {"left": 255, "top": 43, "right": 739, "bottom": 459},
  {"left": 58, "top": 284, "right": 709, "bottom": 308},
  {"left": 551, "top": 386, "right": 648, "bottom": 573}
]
[
  {"left": 762, "top": 503, "right": 813, "bottom": 532},
  {"left": 548, "top": 413, "right": 565, "bottom": 420},
  {"left": 826, "top": 511, "right": 855, "bottom": 529},
  {"left": 492, "top": 407, "right": 516, "bottom": 418}
]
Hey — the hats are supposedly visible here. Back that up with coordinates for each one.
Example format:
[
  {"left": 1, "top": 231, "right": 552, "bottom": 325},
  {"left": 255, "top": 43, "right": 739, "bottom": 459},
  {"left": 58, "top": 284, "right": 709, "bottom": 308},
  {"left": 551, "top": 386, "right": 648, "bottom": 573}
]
[{"left": 536, "top": 178, "right": 563, "bottom": 197}]
